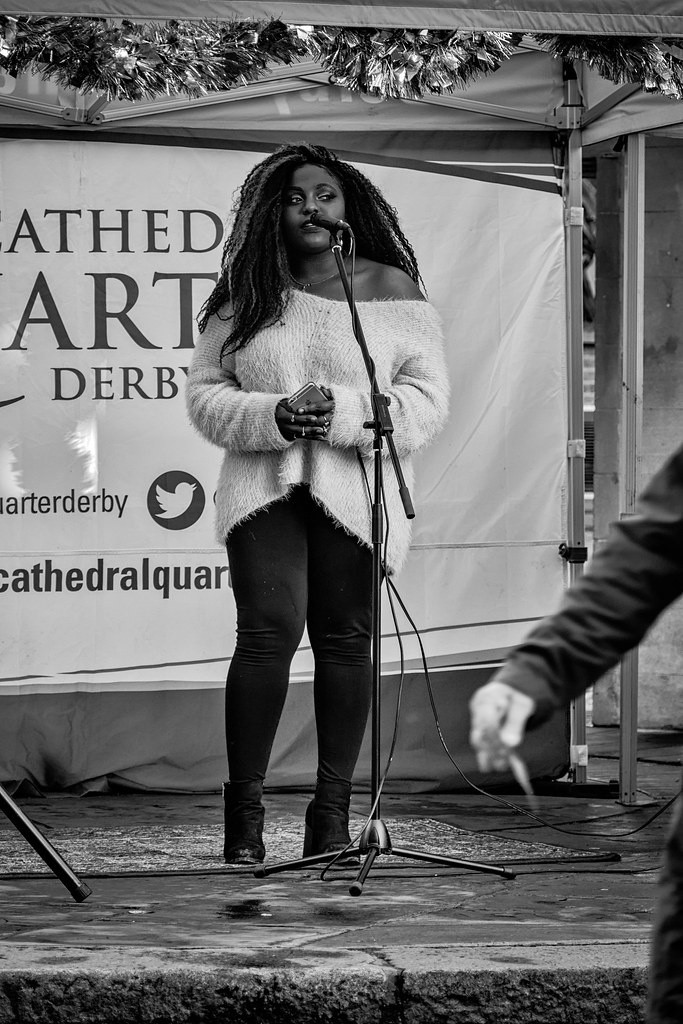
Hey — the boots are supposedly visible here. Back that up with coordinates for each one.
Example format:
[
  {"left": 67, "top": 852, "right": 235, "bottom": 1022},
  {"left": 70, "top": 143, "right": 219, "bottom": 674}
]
[
  {"left": 302, "top": 781, "right": 361, "bottom": 865},
  {"left": 223, "top": 779, "right": 266, "bottom": 864}
]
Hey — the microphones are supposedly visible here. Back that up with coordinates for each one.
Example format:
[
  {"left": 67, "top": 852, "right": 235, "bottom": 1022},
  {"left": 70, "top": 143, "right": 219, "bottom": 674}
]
[{"left": 310, "top": 213, "right": 350, "bottom": 232}]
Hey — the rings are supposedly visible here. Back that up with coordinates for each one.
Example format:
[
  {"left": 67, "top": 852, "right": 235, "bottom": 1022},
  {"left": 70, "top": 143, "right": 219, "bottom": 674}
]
[
  {"left": 323, "top": 425, "right": 327, "bottom": 436},
  {"left": 302, "top": 426, "right": 305, "bottom": 437},
  {"left": 293, "top": 434, "right": 296, "bottom": 440},
  {"left": 291, "top": 413, "right": 295, "bottom": 422},
  {"left": 323, "top": 415, "right": 330, "bottom": 426}
]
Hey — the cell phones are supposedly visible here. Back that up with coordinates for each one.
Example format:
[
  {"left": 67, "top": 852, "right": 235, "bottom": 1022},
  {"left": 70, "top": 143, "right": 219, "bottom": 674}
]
[{"left": 287, "top": 382, "right": 329, "bottom": 413}]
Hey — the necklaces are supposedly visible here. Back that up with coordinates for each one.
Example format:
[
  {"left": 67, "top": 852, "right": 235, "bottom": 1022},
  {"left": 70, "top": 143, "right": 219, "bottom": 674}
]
[{"left": 288, "top": 252, "right": 347, "bottom": 292}]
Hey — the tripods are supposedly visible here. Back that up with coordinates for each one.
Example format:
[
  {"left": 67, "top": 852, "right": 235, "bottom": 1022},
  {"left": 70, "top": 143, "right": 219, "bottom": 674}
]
[{"left": 254, "top": 231, "right": 517, "bottom": 897}]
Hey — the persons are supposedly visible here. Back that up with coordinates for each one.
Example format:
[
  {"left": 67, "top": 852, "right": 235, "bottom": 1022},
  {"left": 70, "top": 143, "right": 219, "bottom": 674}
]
[
  {"left": 469, "top": 438, "right": 683, "bottom": 1024},
  {"left": 187, "top": 141, "right": 450, "bottom": 865}
]
